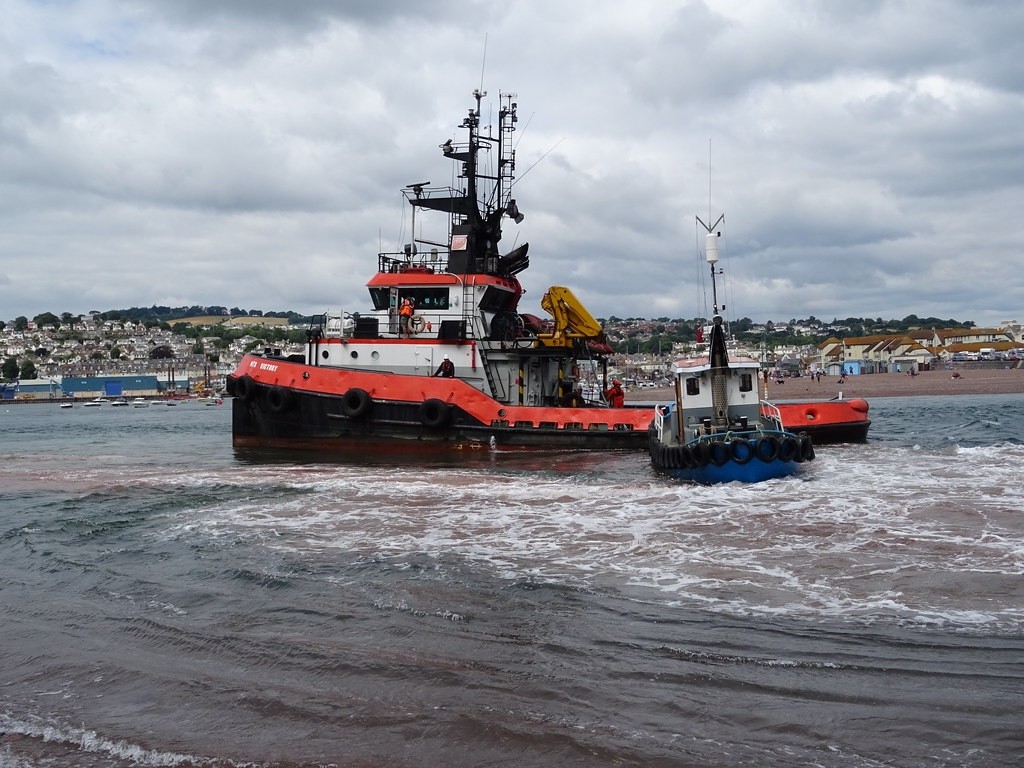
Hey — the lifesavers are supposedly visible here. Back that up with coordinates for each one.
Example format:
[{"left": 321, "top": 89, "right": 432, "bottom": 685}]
[
  {"left": 646, "top": 427, "right": 815, "bottom": 470},
  {"left": 234, "top": 373, "right": 254, "bottom": 400},
  {"left": 265, "top": 386, "right": 288, "bottom": 413},
  {"left": 419, "top": 398, "right": 447, "bottom": 427},
  {"left": 408, "top": 314, "right": 425, "bottom": 333},
  {"left": 340, "top": 388, "right": 371, "bottom": 416}
]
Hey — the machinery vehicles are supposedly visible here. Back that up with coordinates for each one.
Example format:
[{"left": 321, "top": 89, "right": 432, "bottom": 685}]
[
  {"left": 49, "top": 379, "right": 74, "bottom": 398},
  {"left": 193, "top": 376, "right": 208, "bottom": 393}
]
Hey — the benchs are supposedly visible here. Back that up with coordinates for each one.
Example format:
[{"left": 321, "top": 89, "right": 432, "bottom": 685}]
[
  {"left": 287, "top": 354, "right": 305, "bottom": 365},
  {"left": 534, "top": 396, "right": 566, "bottom": 408}
]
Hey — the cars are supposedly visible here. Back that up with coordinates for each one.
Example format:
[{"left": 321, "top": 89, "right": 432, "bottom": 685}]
[
  {"left": 952, "top": 353, "right": 966, "bottom": 361},
  {"left": 1008, "top": 350, "right": 1024, "bottom": 360},
  {"left": 967, "top": 354, "right": 979, "bottom": 361},
  {"left": 980, "top": 352, "right": 992, "bottom": 360},
  {"left": 990, "top": 353, "right": 1006, "bottom": 361}
]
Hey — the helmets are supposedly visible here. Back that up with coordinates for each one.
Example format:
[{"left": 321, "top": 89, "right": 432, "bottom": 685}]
[
  {"left": 444, "top": 355, "right": 449, "bottom": 359},
  {"left": 614, "top": 379, "right": 621, "bottom": 385}
]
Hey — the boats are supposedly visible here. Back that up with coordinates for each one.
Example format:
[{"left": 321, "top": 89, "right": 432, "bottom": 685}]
[
  {"left": 206, "top": 398, "right": 224, "bottom": 406},
  {"left": 83, "top": 402, "right": 101, "bottom": 407},
  {"left": 583, "top": 388, "right": 600, "bottom": 395},
  {"left": 199, "top": 398, "right": 210, "bottom": 402},
  {"left": 111, "top": 398, "right": 129, "bottom": 406},
  {"left": 151, "top": 400, "right": 171, "bottom": 405},
  {"left": 133, "top": 398, "right": 145, "bottom": 405},
  {"left": 646, "top": 214, "right": 815, "bottom": 485},
  {"left": 93, "top": 397, "right": 107, "bottom": 402},
  {"left": 60, "top": 403, "right": 72, "bottom": 408},
  {"left": 225, "top": 88, "right": 871, "bottom": 463}
]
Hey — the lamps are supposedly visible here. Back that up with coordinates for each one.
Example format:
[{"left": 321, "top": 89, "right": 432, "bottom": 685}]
[
  {"left": 703, "top": 416, "right": 712, "bottom": 431},
  {"left": 740, "top": 415, "right": 748, "bottom": 430}
]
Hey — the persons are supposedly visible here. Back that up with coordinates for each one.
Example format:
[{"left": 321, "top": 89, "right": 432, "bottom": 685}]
[
  {"left": 773, "top": 371, "right": 778, "bottom": 384},
  {"left": 810, "top": 372, "right": 820, "bottom": 383},
  {"left": 849, "top": 366, "right": 854, "bottom": 376},
  {"left": 840, "top": 370, "right": 848, "bottom": 380},
  {"left": 430, "top": 354, "right": 455, "bottom": 378},
  {"left": 399, "top": 297, "right": 415, "bottom": 340},
  {"left": 604, "top": 380, "right": 625, "bottom": 408},
  {"left": 910, "top": 367, "right": 914, "bottom": 378},
  {"left": 951, "top": 372, "right": 961, "bottom": 379},
  {"left": 575, "top": 386, "right": 585, "bottom": 408}
]
